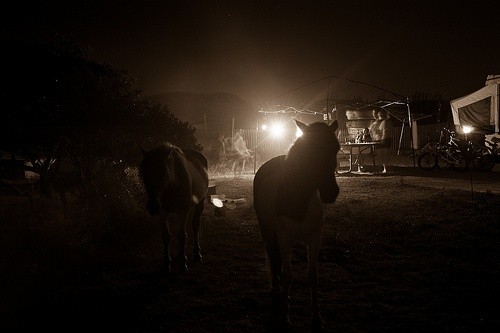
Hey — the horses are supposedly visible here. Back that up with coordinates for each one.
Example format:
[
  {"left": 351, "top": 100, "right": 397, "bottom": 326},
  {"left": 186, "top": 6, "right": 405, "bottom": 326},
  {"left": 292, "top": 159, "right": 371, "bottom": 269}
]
[
  {"left": 134, "top": 138, "right": 212, "bottom": 283},
  {"left": 250, "top": 117, "right": 342, "bottom": 333}
]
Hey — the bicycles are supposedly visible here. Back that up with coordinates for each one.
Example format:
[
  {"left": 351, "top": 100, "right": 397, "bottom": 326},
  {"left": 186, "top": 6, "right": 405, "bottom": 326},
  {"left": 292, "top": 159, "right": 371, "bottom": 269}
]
[{"left": 417, "top": 127, "right": 497, "bottom": 174}]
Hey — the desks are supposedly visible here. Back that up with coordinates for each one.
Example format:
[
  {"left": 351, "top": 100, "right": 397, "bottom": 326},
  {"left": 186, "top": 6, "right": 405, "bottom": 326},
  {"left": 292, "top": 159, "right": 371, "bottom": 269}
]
[{"left": 334, "top": 142, "right": 384, "bottom": 173}]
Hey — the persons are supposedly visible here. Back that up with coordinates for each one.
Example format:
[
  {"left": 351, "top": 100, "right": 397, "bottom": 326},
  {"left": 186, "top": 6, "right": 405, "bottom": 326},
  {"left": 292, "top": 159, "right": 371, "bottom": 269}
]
[{"left": 361, "top": 109, "right": 392, "bottom": 174}]
